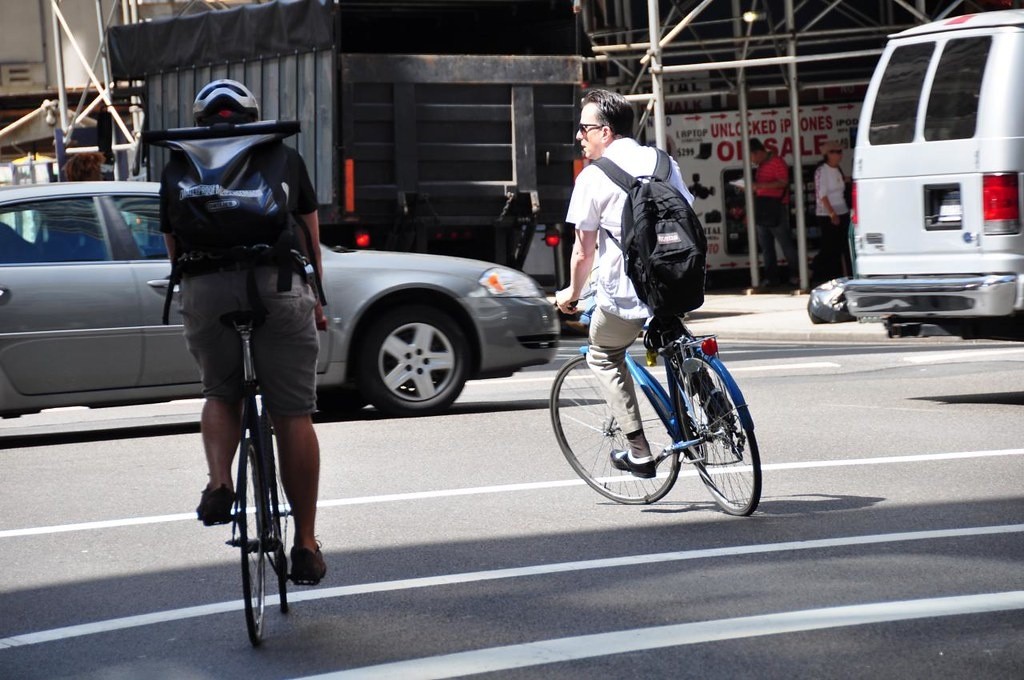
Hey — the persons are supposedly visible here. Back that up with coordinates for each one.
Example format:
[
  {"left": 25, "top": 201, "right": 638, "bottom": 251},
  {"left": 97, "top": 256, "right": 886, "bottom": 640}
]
[
  {"left": 552, "top": 87, "right": 711, "bottom": 479},
  {"left": 812, "top": 140, "right": 854, "bottom": 286},
  {"left": 735, "top": 139, "right": 799, "bottom": 290},
  {"left": 61, "top": 151, "right": 106, "bottom": 182},
  {"left": 159, "top": 80, "right": 328, "bottom": 584}
]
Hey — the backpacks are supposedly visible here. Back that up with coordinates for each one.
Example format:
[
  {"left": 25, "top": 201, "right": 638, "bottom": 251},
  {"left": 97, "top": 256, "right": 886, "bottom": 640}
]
[
  {"left": 592, "top": 147, "right": 708, "bottom": 317},
  {"left": 141, "top": 120, "right": 328, "bottom": 325}
]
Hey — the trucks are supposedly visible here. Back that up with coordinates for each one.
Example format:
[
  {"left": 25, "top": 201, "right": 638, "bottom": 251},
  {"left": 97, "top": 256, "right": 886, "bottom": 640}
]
[{"left": 98, "top": 0, "right": 582, "bottom": 270}]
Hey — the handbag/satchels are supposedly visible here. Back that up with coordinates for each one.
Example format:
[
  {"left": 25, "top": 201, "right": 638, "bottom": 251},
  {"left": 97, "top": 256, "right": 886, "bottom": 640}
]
[
  {"left": 837, "top": 165, "right": 852, "bottom": 209},
  {"left": 753, "top": 196, "right": 784, "bottom": 227}
]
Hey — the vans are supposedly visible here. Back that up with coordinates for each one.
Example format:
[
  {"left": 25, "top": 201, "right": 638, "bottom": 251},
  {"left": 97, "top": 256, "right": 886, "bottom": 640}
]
[{"left": 844, "top": 10, "right": 1024, "bottom": 338}]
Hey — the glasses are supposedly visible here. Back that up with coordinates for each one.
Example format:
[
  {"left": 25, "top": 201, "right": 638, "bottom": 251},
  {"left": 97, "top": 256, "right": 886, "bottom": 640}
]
[
  {"left": 831, "top": 150, "right": 842, "bottom": 153},
  {"left": 579, "top": 123, "right": 598, "bottom": 132}
]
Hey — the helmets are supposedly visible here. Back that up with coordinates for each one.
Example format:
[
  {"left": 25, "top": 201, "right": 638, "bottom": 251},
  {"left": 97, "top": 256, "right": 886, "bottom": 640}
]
[{"left": 193, "top": 79, "right": 258, "bottom": 117}]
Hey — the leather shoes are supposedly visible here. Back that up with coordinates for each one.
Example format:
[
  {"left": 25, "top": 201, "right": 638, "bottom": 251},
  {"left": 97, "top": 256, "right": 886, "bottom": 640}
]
[
  {"left": 704, "top": 389, "right": 730, "bottom": 418},
  {"left": 609, "top": 449, "right": 656, "bottom": 478}
]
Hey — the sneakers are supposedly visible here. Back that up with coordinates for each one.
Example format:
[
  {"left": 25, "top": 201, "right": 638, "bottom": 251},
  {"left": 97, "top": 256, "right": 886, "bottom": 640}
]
[
  {"left": 197, "top": 486, "right": 234, "bottom": 526},
  {"left": 290, "top": 546, "right": 326, "bottom": 586}
]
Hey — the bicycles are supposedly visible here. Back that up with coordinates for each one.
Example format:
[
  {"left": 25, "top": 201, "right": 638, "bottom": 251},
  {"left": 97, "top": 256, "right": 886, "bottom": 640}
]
[
  {"left": 549, "top": 291, "right": 761, "bottom": 515},
  {"left": 166, "top": 271, "right": 328, "bottom": 647}
]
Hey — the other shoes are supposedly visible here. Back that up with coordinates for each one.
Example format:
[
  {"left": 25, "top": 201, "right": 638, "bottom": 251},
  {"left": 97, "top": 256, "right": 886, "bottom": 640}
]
[
  {"left": 789, "top": 276, "right": 800, "bottom": 288},
  {"left": 761, "top": 280, "right": 773, "bottom": 288}
]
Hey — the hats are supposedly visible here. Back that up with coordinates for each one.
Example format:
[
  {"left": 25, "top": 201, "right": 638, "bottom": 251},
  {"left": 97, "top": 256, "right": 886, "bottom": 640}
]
[{"left": 821, "top": 141, "right": 846, "bottom": 156}]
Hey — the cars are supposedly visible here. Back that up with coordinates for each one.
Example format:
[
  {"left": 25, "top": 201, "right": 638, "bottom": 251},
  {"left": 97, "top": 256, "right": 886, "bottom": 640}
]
[{"left": 0, "top": 181, "right": 561, "bottom": 415}]
